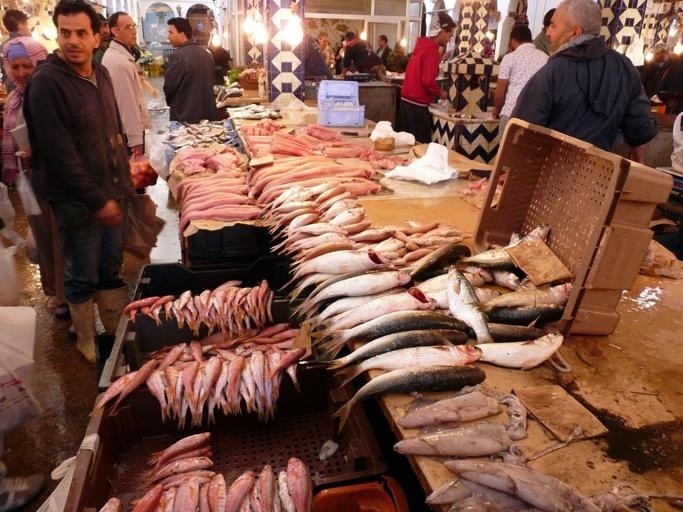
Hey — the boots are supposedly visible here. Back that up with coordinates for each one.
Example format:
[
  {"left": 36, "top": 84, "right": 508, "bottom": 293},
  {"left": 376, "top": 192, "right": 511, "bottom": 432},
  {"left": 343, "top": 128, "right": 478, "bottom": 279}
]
[
  {"left": 67, "top": 297, "right": 98, "bottom": 363},
  {"left": 95, "top": 282, "right": 131, "bottom": 335}
]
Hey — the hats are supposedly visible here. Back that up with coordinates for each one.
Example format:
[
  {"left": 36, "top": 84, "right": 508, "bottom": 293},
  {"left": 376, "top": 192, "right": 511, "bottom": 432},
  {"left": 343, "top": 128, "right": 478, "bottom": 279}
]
[
  {"left": 5, "top": 43, "right": 28, "bottom": 60},
  {"left": 439, "top": 13, "right": 454, "bottom": 28}
]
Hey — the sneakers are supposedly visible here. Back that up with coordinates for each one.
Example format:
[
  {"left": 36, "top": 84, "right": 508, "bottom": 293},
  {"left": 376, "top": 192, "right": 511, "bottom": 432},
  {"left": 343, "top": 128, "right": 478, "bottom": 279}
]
[
  {"left": 55, "top": 303, "right": 77, "bottom": 339},
  {"left": 0, "top": 472, "right": 45, "bottom": 510}
]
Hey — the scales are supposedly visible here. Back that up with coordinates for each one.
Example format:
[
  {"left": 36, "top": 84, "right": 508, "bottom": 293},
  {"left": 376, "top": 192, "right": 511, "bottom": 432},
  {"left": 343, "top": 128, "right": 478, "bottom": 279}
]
[{"left": 656, "top": 167, "right": 683, "bottom": 201}]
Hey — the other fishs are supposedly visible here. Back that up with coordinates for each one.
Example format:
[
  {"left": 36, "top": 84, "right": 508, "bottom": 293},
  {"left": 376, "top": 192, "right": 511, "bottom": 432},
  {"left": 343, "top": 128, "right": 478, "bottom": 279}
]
[
  {"left": 428, "top": 224, "right": 573, "bottom": 370},
  {"left": 126, "top": 430, "right": 315, "bottom": 512},
  {"left": 162, "top": 103, "right": 283, "bottom": 149},
  {"left": 88, "top": 278, "right": 307, "bottom": 431},
  {"left": 260, "top": 180, "right": 485, "bottom": 435},
  {"left": 258, "top": 178, "right": 603, "bottom": 512}
]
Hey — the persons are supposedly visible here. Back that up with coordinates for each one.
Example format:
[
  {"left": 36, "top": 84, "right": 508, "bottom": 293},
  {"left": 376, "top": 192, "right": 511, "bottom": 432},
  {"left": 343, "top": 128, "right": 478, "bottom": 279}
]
[
  {"left": 302, "top": 25, "right": 411, "bottom": 98},
  {"left": 641, "top": 42, "right": 682, "bottom": 108},
  {"left": 94, "top": 11, "right": 157, "bottom": 194},
  {"left": 531, "top": 7, "right": 556, "bottom": 55},
  {"left": 490, "top": 22, "right": 550, "bottom": 142},
  {"left": 164, "top": 17, "right": 229, "bottom": 133},
  {"left": 1, "top": 8, "right": 70, "bottom": 318},
  {"left": 397, "top": 10, "right": 456, "bottom": 143},
  {"left": 22, "top": 1, "right": 135, "bottom": 367},
  {"left": 509, "top": 0, "right": 658, "bottom": 165}
]
